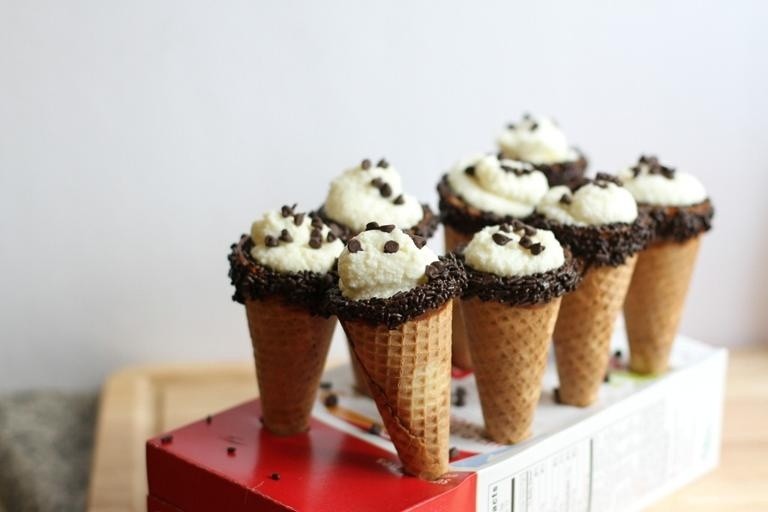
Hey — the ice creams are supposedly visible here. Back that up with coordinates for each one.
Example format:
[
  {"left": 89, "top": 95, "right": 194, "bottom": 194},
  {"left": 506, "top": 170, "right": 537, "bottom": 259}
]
[{"left": 228, "top": 113, "right": 714, "bottom": 481}]
